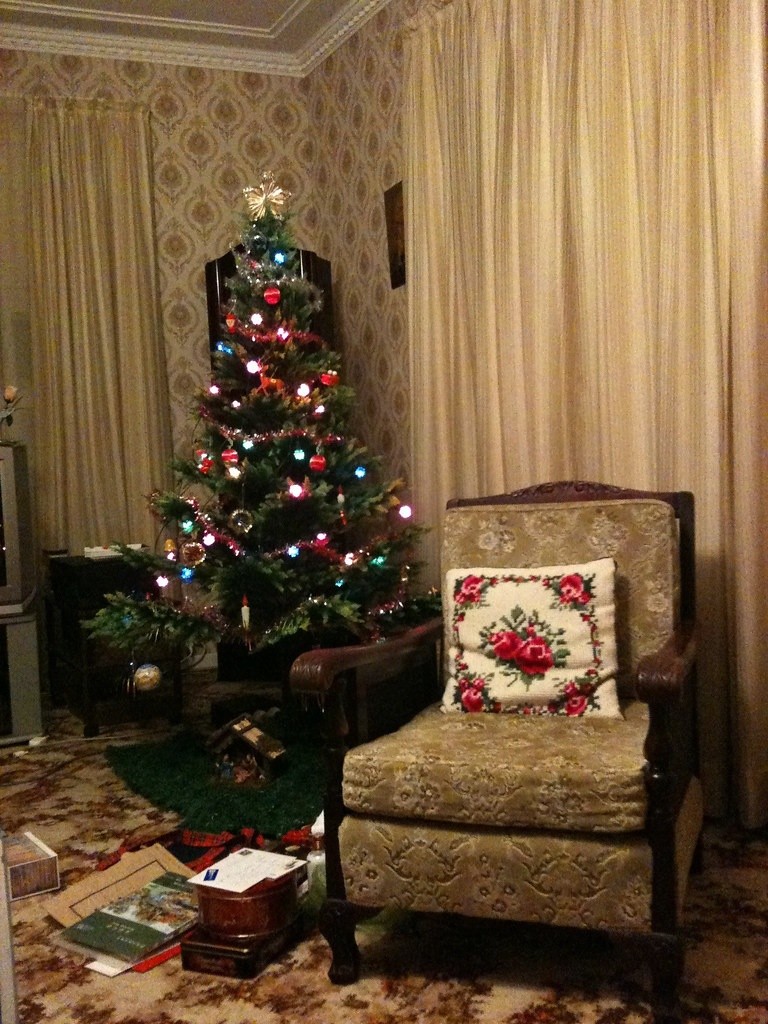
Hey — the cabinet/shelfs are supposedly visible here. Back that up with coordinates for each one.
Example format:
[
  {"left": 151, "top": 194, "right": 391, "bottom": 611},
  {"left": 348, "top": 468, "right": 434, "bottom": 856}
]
[{"left": 42, "top": 553, "right": 187, "bottom": 736}]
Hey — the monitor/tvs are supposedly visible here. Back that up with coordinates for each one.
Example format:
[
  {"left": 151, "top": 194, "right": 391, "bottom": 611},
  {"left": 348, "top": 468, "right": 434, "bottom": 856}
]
[{"left": 0, "top": 440, "right": 37, "bottom": 618}]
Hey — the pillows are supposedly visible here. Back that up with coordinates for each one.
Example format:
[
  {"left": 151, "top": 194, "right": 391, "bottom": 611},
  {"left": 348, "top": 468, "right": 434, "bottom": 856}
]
[{"left": 441, "top": 557, "right": 626, "bottom": 718}]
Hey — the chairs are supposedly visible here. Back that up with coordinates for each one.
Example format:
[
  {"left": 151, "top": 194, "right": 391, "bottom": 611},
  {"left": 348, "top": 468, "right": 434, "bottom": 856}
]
[{"left": 288, "top": 480, "right": 706, "bottom": 1024}]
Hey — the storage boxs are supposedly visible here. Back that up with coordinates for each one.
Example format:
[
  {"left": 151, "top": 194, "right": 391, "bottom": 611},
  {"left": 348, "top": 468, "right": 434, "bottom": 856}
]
[
  {"left": 179, "top": 929, "right": 279, "bottom": 979},
  {"left": 230, "top": 715, "right": 287, "bottom": 777},
  {"left": 4, "top": 831, "right": 61, "bottom": 900}
]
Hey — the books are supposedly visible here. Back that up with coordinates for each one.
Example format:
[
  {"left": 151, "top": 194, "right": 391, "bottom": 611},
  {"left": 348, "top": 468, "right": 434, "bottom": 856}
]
[{"left": 44, "top": 842, "right": 199, "bottom": 978}]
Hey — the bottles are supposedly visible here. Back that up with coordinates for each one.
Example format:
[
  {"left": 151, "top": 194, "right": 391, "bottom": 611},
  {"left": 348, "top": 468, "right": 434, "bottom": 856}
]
[{"left": 306, "top": 840, "right": 327, "bottom": 916}]
